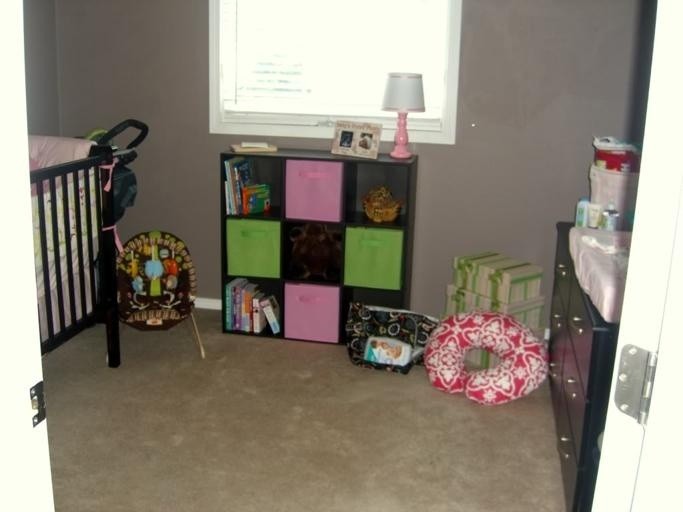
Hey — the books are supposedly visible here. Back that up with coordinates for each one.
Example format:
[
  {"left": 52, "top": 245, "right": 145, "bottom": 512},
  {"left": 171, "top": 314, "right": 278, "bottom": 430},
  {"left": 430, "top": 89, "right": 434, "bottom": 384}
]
[
  {"left": 225, "top": 278, "right": 281, "bottom": 334},
  {"left": 240, "top": 140, "right": 268, "bottom": 148},
  {"left": 229, "top": 143, "right": 278, "bottom": 153},
  {"left": 223, "top": 157, "right": 271, "bottom": 215}
]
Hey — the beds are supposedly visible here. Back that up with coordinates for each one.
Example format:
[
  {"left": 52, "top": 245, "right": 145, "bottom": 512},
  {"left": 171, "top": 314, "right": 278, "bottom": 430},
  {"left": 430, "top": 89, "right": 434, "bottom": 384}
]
[{"left": 30, "top": 133, "right": 121, "bottom": 368}]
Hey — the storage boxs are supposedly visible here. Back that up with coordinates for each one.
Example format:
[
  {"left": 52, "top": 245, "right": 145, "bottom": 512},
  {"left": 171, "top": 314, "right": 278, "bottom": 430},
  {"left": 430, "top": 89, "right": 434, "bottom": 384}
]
[{"left": 445, "top": 251, "right": 542, "bottom": 370}]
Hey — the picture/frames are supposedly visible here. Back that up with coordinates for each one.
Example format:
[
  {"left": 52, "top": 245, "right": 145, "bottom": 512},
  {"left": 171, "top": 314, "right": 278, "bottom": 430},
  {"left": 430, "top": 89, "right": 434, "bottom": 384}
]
[{"left": 331, "top": 120, "right": 383, "bottom": 159}]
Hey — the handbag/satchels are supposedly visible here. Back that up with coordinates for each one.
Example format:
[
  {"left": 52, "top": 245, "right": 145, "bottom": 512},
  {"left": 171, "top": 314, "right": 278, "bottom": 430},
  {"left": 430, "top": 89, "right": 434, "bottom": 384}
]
[{"left": 344, "top": 300, "right": 440, "bottom": 376}]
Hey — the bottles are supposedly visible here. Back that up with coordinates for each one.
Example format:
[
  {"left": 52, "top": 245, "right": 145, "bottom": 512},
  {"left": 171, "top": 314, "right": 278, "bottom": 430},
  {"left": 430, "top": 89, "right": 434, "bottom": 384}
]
[
  {"left": 574, "top": 196, "right": 589, "bottom": 227},
  {"left": 601, "top": 203, "right": 620, "bottom": 230}
]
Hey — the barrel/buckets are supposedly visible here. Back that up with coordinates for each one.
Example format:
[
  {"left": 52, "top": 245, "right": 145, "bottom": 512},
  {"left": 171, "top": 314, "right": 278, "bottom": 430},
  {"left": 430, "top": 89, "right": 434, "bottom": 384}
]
[{"left": 591, "top": 168, "right": 640, "bottom": 229}]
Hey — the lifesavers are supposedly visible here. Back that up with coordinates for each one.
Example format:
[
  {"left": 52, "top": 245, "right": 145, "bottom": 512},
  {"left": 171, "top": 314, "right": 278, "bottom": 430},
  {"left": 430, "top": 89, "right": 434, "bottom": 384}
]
[{"left": 423, "top": 310, "right": 548, "bottom": 405}]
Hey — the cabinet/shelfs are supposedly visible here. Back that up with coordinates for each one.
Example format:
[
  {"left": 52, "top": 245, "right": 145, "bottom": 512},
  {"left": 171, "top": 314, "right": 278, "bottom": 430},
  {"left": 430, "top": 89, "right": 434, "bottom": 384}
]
[
  {"left": 220, "top": 148, "right": 419, "bottom": 345},
  {"left": 544, "top": 221, "right": 633, "bottom": 511}
]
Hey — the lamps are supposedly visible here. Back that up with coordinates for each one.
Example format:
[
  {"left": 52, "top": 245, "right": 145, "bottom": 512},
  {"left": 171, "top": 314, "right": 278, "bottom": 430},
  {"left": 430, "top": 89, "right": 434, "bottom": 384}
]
[{"left": 381, "top": 73, "right": 425, "bottom": 160}]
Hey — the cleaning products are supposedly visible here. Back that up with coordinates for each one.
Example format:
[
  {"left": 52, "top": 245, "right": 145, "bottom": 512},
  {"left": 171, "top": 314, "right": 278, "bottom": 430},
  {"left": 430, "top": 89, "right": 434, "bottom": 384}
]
[{"left": 598, "top": 198, "right": 622, "bottom": 229}]
